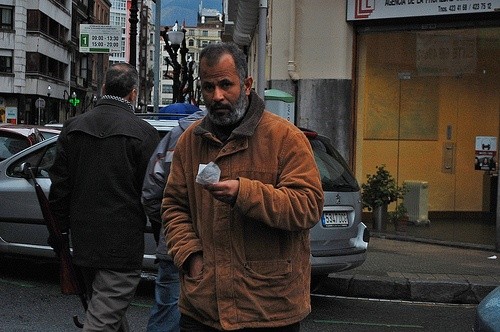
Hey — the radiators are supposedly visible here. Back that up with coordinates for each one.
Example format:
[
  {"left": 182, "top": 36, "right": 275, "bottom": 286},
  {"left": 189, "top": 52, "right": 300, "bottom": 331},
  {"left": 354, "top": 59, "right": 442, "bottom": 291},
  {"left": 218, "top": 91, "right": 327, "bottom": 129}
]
[{"left": 401, "top": 180, "right": 430, "bottom": 224}]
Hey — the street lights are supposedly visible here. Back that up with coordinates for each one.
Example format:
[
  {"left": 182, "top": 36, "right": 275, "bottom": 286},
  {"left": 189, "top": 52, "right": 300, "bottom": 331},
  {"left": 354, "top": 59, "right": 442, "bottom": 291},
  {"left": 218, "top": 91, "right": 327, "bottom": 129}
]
[
  {"left": 167, "top": 20, "right": 185, "bottom": 104},
  {"left": 46, "top": 85, "right": 52, "bottom": 122},
  {"left": 72, "top": 91, "right": 77, "bottom": 116}
]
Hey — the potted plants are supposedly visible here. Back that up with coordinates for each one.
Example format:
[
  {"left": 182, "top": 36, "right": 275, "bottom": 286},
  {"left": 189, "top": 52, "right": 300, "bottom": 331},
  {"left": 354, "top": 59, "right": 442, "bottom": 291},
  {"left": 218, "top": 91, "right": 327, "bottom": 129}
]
[
  {"left": 360, "top": 165, "right": 407, "bottom": 232},
  {"left": 387, "top": 204, "right": 409, "bottom": 232}
]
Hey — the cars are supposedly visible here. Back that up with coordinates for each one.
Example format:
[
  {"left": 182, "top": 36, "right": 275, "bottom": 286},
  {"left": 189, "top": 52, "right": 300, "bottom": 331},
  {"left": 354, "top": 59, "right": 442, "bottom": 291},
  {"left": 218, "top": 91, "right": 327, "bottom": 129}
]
[
  {"left": 0, "top": 121, "right": 64, "bottom": 163},
  {"left": 1, "top": 112, "right": 372, "bottom": 291}
]
